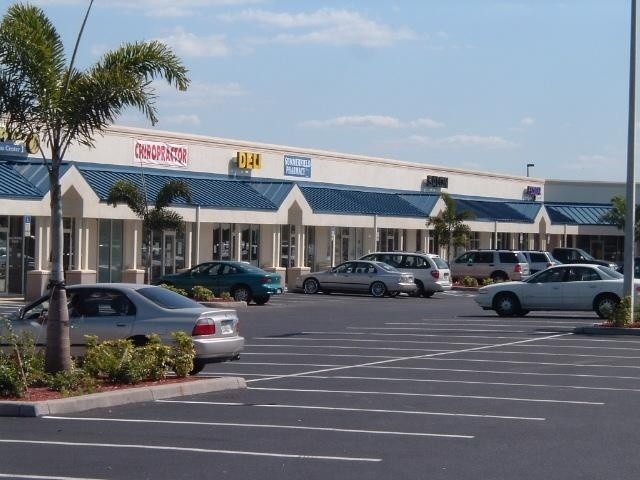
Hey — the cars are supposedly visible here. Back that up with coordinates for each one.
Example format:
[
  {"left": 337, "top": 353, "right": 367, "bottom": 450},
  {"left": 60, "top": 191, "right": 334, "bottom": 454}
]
[
  {"left": 0, "top": 241, "right": 183, "bottom": 277},
  {"left": 294, "top": 258, "right": 419, "bottom": 297},
  {"left": 1, "top": 281, "right": 245, "bottom": 377},
  {"left": 155, "top": 260, "right": 284, "bottom": 306},
  {"left": 620, "top": 255, "right": 640, "bottom": 278},
  {"left": 472, "top": 260, "right": 640, "bottom": 319}
]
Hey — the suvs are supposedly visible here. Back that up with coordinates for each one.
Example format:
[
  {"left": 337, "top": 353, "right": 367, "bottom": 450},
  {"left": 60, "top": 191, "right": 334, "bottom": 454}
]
[
  {"left": 344, "top": 248, "right": 566, "bottom": 299},
  {"left": 554, "top": 246, "right": 620, "bottom": 272}
]
